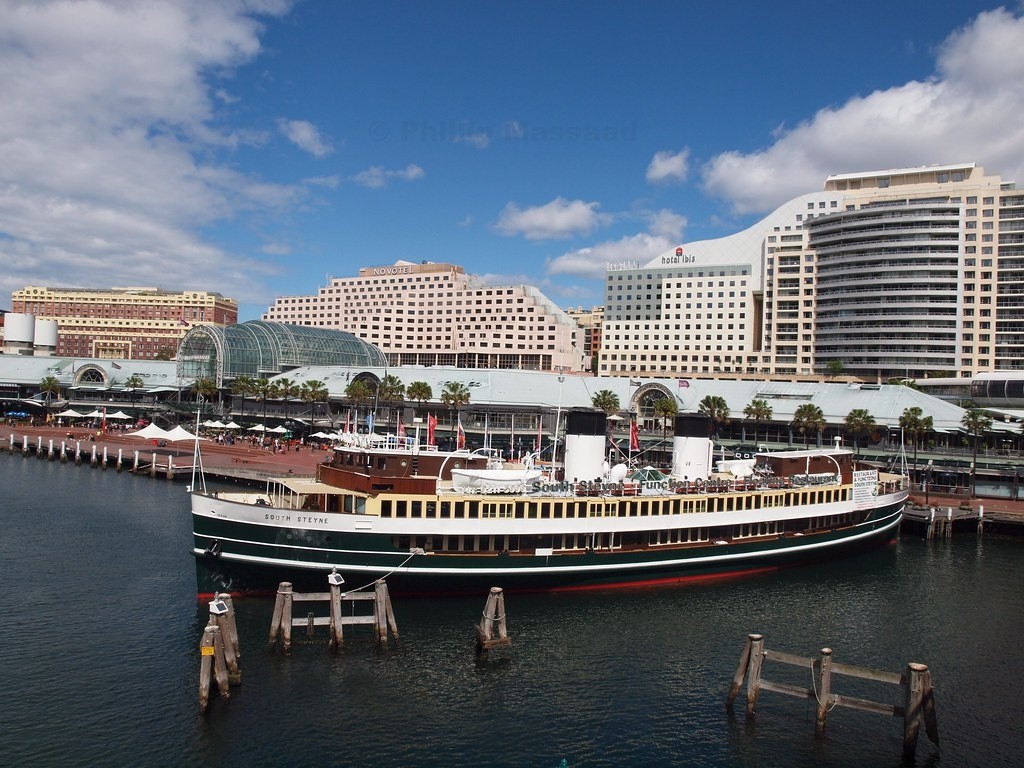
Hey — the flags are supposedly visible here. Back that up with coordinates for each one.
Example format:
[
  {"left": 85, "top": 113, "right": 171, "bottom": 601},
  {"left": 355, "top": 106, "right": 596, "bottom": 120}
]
[
  {"left": 352, "top": 416, "right": 358, "bottom": 433},
  {"left": 678, "top": 379, "right": 690, "bottom": 388},
  {"left": 398, "top": 419, "right": 407, "bottom": 443},
  {"left": 630, "top": 379, "right": 641, "bottom": 386},
  {"left": 428, "top": 415, "right": 438, "bottom": 446},
  {"left": 629, "top": 420, "right": 640, "bottom": 451},
  {"left": 458, "top": 420, "right": 465, "bottom": 449},
  {"left": 343, "top": 415, "right": 349, "bottom": 432}
]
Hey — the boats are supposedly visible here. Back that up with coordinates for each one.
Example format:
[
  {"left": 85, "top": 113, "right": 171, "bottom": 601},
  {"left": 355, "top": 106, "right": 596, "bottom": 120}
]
[
  {"left": 449, "top": 467, "right": 543, "bottom": 495},
  {"left": 716, "top": 457, "right": 757, "bottom": 473}
]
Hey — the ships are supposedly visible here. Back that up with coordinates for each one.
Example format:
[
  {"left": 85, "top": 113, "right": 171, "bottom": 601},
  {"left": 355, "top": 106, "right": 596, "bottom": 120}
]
[{"left": 185, "top": 407, "right": 909, "bottom": 609}]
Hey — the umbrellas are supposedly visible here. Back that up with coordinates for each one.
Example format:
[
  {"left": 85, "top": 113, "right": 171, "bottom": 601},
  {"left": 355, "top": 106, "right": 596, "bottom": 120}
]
[
  {"left": 7, "top": 411, "right": 28, "bottom": 417},
  {"left": 247, "top": 424, "right": 293, "bottom": 434},
  {"left": 124, "top": 422, "right": 204, "bottom": 456},
  {"left": 54, "top": 409, "right": 133, "bottom": 428},
  {"left": 606, "top": 414, "right": 624, "bottom": 421},
  {"left": 200, "top": 420, "right": 243, "bottom": 429}
]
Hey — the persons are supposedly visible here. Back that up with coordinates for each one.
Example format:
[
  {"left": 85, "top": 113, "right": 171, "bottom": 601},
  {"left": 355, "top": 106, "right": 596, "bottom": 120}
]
[
  {"left": 9, "top": 417, "right": 15, "bottom": 429},
  {"left": 87, "top": 420, "right": 125, "bottom": 434},
  {"left": 30, "top": 415, "right": 42, "bottom": 428},
  {"left": 236, "top": 432, "right": 282, "bottom": 453},
  {"left": 204, "top": 429, "right": 232, "bottom": 446}
]
